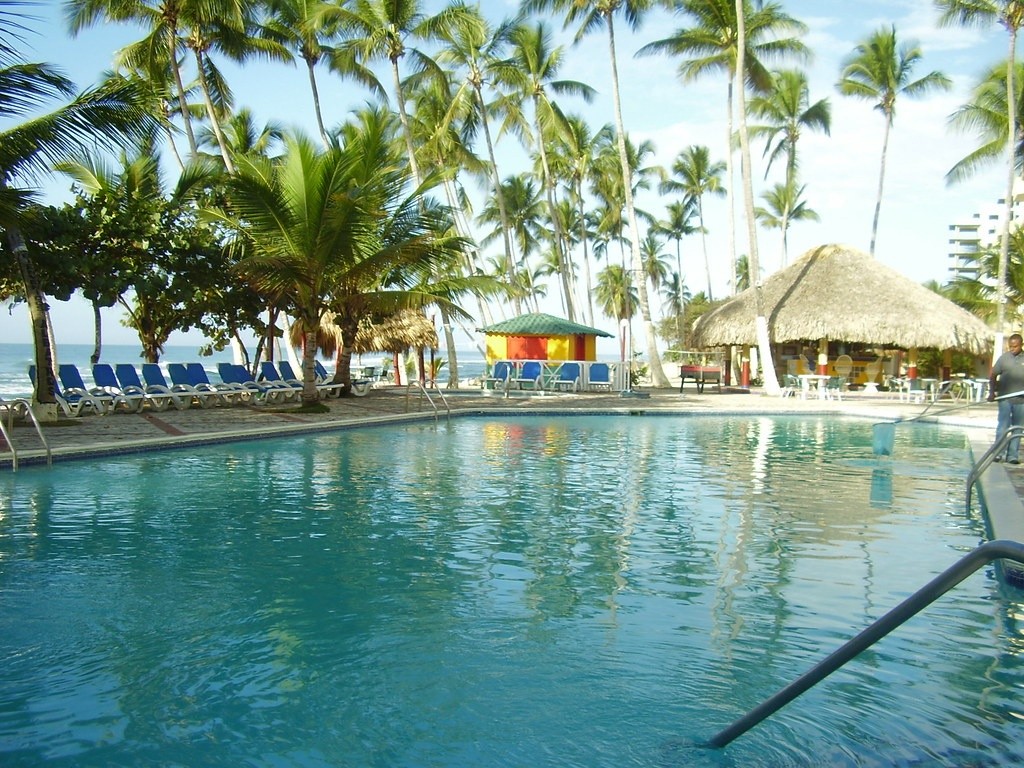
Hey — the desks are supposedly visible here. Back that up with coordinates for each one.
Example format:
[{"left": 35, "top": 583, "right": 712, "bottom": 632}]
[
  {"left": 680, "top": 365, "right": 722, "bottom": 394},
  {"left": 889, "top": 378, "right": 938, "bottom": 404},
  {"left": 797, "top": 375, "right": 832, "bottom": 401}
]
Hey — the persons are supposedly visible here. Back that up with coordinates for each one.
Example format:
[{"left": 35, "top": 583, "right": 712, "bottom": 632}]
[
  {"left": 805, "top": 350, "right": 817, "bottom": 371},
  {"left": 987, "top": 334, "right": 1024, "bottom": 464}
]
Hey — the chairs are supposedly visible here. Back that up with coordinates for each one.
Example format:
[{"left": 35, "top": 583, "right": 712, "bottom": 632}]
[
  {"left": 481, "top": 360, "right": 514, "bottom": 390},
  {"left": 778, "top": 354, "right": 990, "bottom": 409},
  {"left": 587, "top": 363, "right": 611, "bottom": 393},
  {"left": 549, "top": 363, "right": 583, "bottom": 393},
  {"left": 26, "top": 360, "right": 379, "bottom": 416},
  {"left": 511, "top": 361, "right": 544, "bottom": 391}
]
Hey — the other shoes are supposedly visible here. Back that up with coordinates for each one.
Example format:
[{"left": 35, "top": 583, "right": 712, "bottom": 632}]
[
  {"left": 994, "top": 456, "right": 1001, "bottom": 462},
  {"left": 1009, "top": 459, "right": 1019, "bottom": 464}
]
